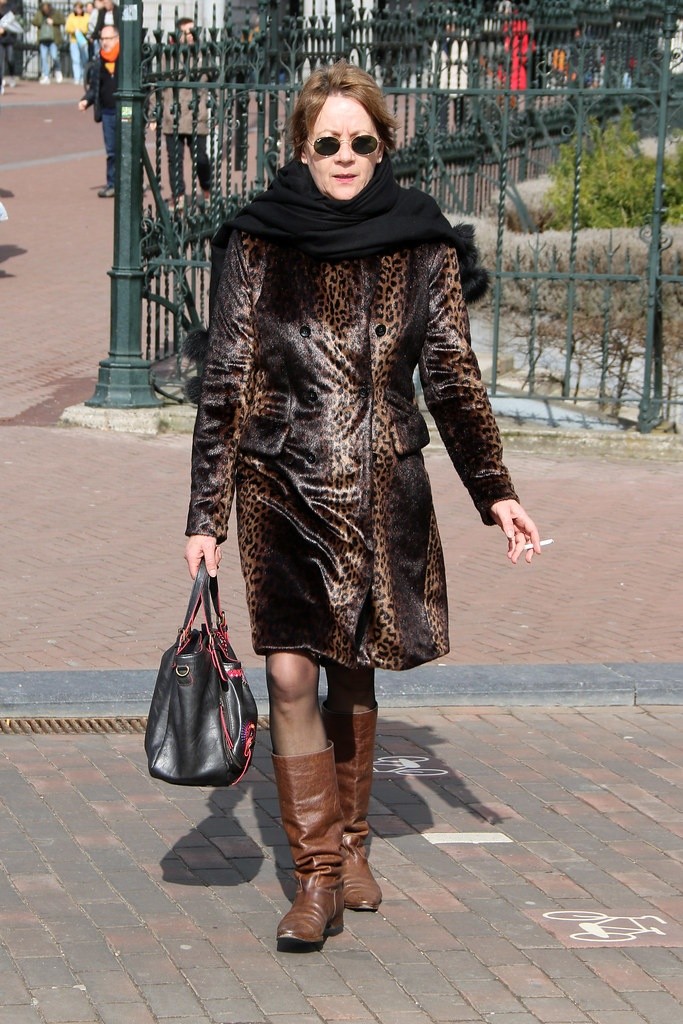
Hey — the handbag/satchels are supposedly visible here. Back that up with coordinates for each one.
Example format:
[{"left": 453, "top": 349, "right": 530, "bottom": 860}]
[
  {"left": 143, "top": 558, "right": 259, "bottom": 787},
  {"left": 39, "top": 20, "right": 54, "bottom": 42}
]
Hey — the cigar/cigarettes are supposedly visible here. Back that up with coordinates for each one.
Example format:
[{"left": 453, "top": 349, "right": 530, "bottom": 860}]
[{"left": 525, "top": 539, "right": 555, "bottom": 550}]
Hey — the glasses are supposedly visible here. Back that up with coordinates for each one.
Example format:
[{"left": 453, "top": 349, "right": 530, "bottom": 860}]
[
  {"left": 99, "top": 34, "right": 118, "bottom": 43},
  {"left": 305, "top": 134, "right": 381, "bottom": 157}
]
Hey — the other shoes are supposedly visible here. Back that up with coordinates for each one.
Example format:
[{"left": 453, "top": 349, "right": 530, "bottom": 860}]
[
  {"left": 40, "top": 76, "right": 50, "bottom": 85},
  {"left": 55, "top": 70, "right": 63, "bottom": 84},
  {"left": 97, "top": 185, "right": 115, "bottom": 197},
  {"left": 204, "top": 189, "right": 211, "bottom": 209},
  {"left": 168, "top": 194, "right": 185, "bottom": 211}
]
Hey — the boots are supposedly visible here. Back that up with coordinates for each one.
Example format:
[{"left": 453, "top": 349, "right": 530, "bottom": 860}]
[
  {"left": 271, "top": 741, "right": 345, "bottom": 944},
  {"left": 321, "top": 694, "right": 382, "bottom": 909}
]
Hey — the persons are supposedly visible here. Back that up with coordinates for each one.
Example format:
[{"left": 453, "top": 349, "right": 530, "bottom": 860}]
[
  {"left": 0, "top": 0, "right": 120, "bottom": 87},
  {"left": 146, "top": 18, "right": 213, "bottom": 210},
  {"left": 78, "top": 26, "right": 150, "bottom": 198},
  {"left": 430, "top": 0, "right": 632, "bottom": 136},
  {"left": 185, "top": 62, "right": 542, "bottom": 947},
  {"left": 241, "top": 13, "right": 292, "bottom": 99}
]
